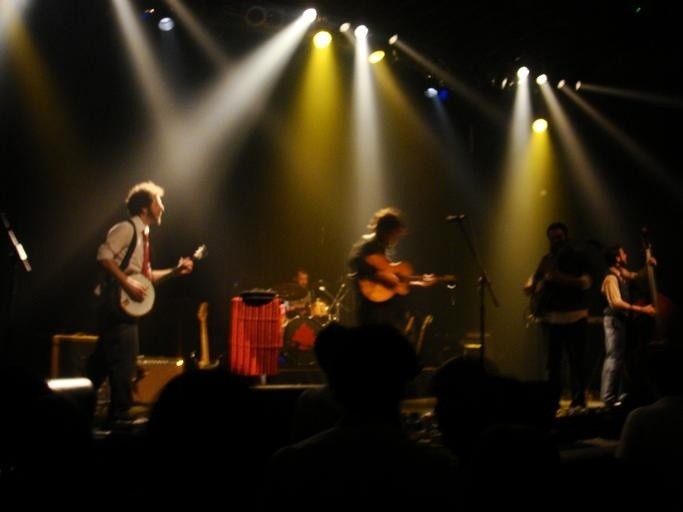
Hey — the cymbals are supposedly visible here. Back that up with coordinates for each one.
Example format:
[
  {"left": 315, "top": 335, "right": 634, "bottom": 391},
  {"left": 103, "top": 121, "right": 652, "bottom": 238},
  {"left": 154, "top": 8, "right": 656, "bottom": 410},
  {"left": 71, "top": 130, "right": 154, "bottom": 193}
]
[{"left": 270, "top": 283, "right": 307, "bottom": 302}]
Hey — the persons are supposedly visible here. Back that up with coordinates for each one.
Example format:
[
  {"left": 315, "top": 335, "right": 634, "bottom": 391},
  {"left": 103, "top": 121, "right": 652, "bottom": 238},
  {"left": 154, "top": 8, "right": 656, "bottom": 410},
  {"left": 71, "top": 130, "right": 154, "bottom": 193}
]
[
  {"left": 287, "top": 271, "right": 311, "bottom": 319},
  {"left": 600, "top": 247, "right": 657, "bottom": 407},
  {"left": 149, "top": 319, "right": 682, "bottom": 511},
  {"left": 347, "top": 208, "right": 434, "bottom": 324},
  {"left": 523, "top": 222, "right": 606, "bottom": 418},
  {"left": 82, "top": 181, "right": 193, "bottom": 436}
]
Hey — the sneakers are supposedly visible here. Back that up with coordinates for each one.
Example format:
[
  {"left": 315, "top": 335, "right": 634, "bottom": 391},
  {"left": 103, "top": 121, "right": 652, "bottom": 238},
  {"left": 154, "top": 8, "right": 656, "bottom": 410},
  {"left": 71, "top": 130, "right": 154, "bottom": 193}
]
[
  {"left": 109, "top": 412, "right": 149, "bottom": 424},
  {"left": 602, "top": 394, "right": 627, "bottom": 407}
]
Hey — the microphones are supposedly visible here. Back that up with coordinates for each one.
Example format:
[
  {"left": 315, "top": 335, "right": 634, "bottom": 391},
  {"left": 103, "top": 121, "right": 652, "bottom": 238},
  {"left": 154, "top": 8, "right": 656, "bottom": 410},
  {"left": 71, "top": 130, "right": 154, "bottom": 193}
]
[{"left": 445, "top": 215, "right": 464, "bottom": 220}]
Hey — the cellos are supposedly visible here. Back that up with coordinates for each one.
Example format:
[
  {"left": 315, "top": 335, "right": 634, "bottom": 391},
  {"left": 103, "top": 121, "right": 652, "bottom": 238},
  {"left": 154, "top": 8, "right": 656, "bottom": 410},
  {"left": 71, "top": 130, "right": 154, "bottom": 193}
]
[{"left": 622, "top": 229, "right": 682, "bottom": 403}]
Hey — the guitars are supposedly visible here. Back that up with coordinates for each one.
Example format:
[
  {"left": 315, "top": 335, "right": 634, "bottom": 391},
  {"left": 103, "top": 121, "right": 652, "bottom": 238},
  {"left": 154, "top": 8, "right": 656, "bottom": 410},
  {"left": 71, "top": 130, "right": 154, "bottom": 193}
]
[
  {"left": 359, "top": 255, "right": 456, "bottom": 302},
  {"left": 190, "top": 303, "right": 219, "bottom": 370}
]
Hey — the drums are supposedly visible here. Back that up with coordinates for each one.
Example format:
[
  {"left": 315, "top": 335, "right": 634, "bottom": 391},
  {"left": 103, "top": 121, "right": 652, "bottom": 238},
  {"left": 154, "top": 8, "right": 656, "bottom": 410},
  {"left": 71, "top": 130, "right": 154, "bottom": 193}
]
[{"left": 283, "top": 318, "right": 322, "bottom": 366}]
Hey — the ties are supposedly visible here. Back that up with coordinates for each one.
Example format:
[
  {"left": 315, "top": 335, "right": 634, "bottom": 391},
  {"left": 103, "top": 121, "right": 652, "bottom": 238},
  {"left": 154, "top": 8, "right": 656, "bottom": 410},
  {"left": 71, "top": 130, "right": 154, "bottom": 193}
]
[{"left": 140, "top": 231, "right": 151, "bottom": 278}]
